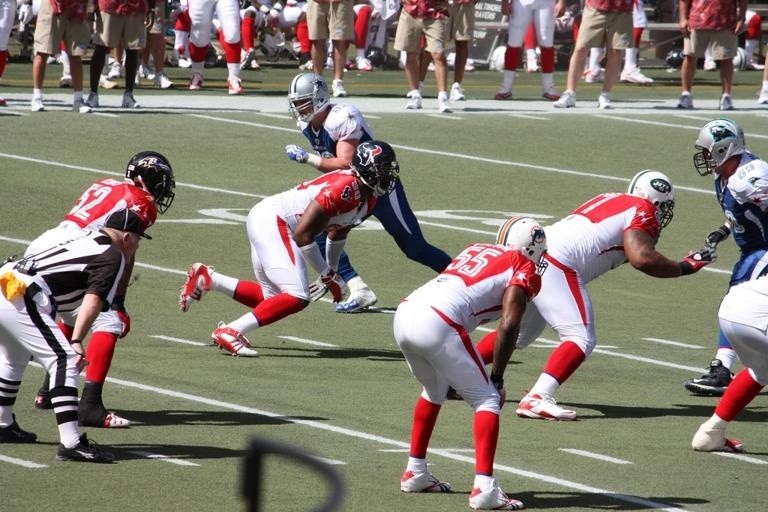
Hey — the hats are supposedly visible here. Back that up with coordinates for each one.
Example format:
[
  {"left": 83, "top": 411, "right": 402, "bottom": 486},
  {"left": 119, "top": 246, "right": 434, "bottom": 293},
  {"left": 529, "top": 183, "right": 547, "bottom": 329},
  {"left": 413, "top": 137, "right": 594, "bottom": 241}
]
[{"left": 103, "top": 208, "right": 152, "bottom": 241}]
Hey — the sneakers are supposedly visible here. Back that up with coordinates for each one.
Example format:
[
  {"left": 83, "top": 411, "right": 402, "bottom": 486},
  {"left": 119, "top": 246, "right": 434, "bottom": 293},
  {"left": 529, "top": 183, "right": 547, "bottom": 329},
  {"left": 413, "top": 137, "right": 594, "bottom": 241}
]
[
  {"left": 407, "top": 89, "right": 422, "bottom": 109},
  {"left": 583, "top": 66, "right": 607, "bottom": 83},
  {"left": 543, "top": 87, "right": 561, "bottom": 101},
  {"left": 677, "top": 93, "right": 695, "bottom": 109},
  {"left": 401, "top": 462, "right": 451, "bottom": 493},
  {"left": 692, "top": 423, "right": 743, "bottom": 452},
  {"left": 554, "top": 90, "right": 576, "bottom": 107},
  {"left": 464, "top": 60, "right": 474, "bottom": 71},
  {"left": 0, "top": 387, "right": 132, "bottom": 463},
  {"left": 322, "top": 57, "right": 335, "bottom": 69},
  {"left": 703, "top": 58, "right": 717, "bottom": 70},
  {"left": 516, "top": 390, "right": 576, "bottom": 421},
  {"left": 354, "top": 56, "right": 372, "bottom": 71},
  {"left": 758, "top": 86, "right": 767, "bottom": 104},
  {"left": 334, "top": 286, "right": 377, "bottom": 313},
  {"left": 211, "top": 321, "right": 258, "bottom": 358},
  {"left": 684, "top": 359, "right": 737, "bottom": 396},
  {"left": 438, "top": 90, "right": 452, "bottom": 111},
  {"left": 526, "top": 50, "right": 541, "bottom": 72},
  {"left": 619, "top": 66, "right": 655, "bottom": 85},
  {"left": 494, "top": 89, "right": 515, "bottom": 101},
  {"left": 596, "top": 91, "right": 611, "bottom": 108},
  {"left": 469, "top": 478, "right": 524, "bottom": 510},
  {"left": 300, "top": 59, "right": 314, "bottom": 71},
  {"left": 449, "top": 83, "right": 466, "bottom": 101},
  {"left": 29, "top": 47, "right": 260, "bottom": 112},
  {"left": 331, "top": 77, "right": 347, "bottom": 98},
  {"left": 426, "top": 62, "right": 435, "bottom": 71},
  {"left": 178, "top": 263, "right": 215, "bottom": 312},
  {"left": 720, "top": 92, "right": 734, "bottom": 111},
  {"left": 748, "top": 59, "right": 766, "bottom": 69}
]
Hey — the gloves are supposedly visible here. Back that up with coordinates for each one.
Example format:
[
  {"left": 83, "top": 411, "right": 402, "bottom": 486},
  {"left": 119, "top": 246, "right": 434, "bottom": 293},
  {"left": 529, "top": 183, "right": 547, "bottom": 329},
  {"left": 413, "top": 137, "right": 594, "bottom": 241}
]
[
  {"left": 309, "top": 276, "right": 328, "bottom": 302},
  {"left": 284, "top": 145, "right": 309, "bottom": 164},
  {"left": 703, "top": 226, "right": 731, "bottom": 249},
  {"left": 679, "top": 246, "right": 718, "bottom": 276},
  {"left": 320, "top": 269, "right": 349, "bottom": 304}
]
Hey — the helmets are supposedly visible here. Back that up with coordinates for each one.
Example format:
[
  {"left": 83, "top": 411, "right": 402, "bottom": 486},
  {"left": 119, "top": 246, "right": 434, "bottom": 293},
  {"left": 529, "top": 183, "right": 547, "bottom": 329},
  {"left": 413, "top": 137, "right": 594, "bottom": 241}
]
[
  {"left": 260, "top": 30, "right": 284, "bottom": 59},
  {"left": 348, "top": 141, "right": 400, "bottom": 198},
  {"left": 496, "top": 216, "right": 548, "bottom": 278},
  {"left": 624, "top": 168, "right": 675, "bottom": 233},
  {"left": 124, "top": 150, "right": 177, "bottom": 214},
  {"left": 692, "top": 118, "right": 747, "bottom": 176},
  {"left": 289, "top": 74, "right": 330, "bottom": 122}
]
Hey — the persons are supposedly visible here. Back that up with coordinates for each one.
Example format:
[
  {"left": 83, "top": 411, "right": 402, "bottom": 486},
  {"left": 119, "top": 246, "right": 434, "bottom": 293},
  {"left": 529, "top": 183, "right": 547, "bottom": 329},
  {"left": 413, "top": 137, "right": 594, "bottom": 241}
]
[
  {"left": 444, "top": 168, "right": 718, "bottom": 420},
  {"left": 394, "top": 0, "right": 767, "bottom": 114},
  {"left": 681, "top": 118, "right": 767, "bottom": 398},
  {"left": 23, "top": 151, "right": 175, "bottom": 429},
  {"left": 393, "top": 217, "right": 548, "bottom": 509},
  {"left": 283, "top": 71, "right": 454, "bottom": 312},
  {"left": 179, "top": 140, "right": 400, "bottom": 357},
  {"left": 1, "top": 1, "right": 402, "bottom": 113},
  {"left": 0, "top": 206, "right": 153, "bottom": 463},
  {"left": 690, "top": 273, "right": 768, "bottom": 453}
]
